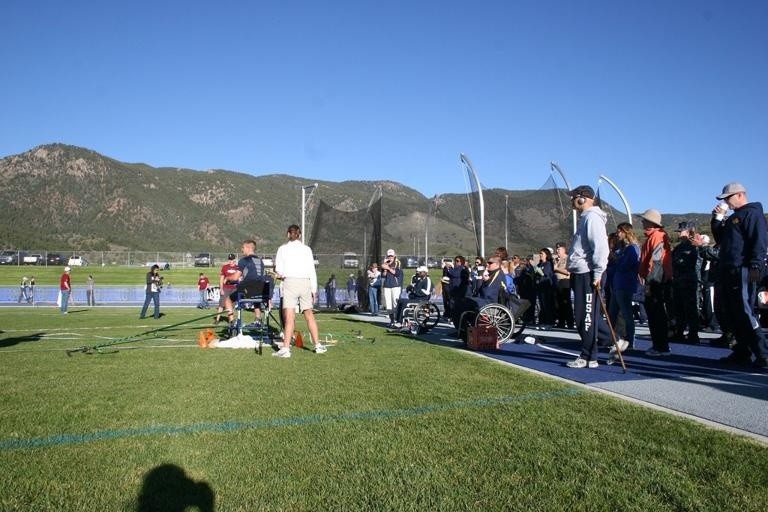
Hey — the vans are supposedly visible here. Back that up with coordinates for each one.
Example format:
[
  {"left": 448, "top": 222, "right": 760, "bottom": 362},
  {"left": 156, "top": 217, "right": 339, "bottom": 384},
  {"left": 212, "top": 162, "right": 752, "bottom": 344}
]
[
  {"left": 312, "top": 254, "right": 321, "bottom": 269},
  {"left": 400, "top": 254, "right": 454, "bottom": 270},
  {"left": 194, "top": 252, "right": 214, "bottom": 267},
  {"left": 0, "top": 250, "right": 24, "bottom": 264},
  {"left": 340, "top": 251, "right": 360, "bottom": 269}
]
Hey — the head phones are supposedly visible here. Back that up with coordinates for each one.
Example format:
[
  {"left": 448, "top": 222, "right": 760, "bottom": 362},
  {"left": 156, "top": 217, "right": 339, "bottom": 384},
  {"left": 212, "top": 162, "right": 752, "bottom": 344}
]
[{"left": 579, "top": 186, "right": 585, "bottom": 205}]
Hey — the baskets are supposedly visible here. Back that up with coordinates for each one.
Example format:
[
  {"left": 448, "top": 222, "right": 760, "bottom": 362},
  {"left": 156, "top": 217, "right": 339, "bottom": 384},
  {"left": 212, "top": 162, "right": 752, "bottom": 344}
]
[{"left": 466, "top": 325, "right": 497, "bottom": 352}]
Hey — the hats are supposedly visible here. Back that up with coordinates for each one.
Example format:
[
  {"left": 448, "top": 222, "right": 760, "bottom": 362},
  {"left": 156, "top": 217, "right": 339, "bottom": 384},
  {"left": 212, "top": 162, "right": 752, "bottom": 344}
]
[
  {"left": 417, "top": 265, "right": 428, "bottom": 273},
  {"left": 227, "top": 253, "right": 235, "bottom": 260},
  {"left": 556, "top": 242, "right": 567, "bottom": 248},
  {"left": 674, "top": 221, "right": 697, "bottom": 232},
  {"left": 387, "top": 249, "right": 395, "bottom": 257},
  {"left": 638, "top": 208, "right": 665, "bottom": 228},
  {"left": 716, "top": 183, "right": 747, "bottom": 200},
  {"left": 567, "top": 185, "right": 595, "bottom": 199},
  {"left": 370, "top": 262, "right": 380, "bottom": 268}
]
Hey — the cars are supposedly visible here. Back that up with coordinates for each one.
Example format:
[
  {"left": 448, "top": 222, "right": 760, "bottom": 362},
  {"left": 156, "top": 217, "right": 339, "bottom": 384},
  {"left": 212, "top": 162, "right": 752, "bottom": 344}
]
[
  {"left": 148, "top": 260, "right": 173, "bottom": 271},
  {"left": 44, "top": 250, "right": 93, "bottom": 268},
  {"left": 260, "top": 255, "right": 274, "bottom": 268}
]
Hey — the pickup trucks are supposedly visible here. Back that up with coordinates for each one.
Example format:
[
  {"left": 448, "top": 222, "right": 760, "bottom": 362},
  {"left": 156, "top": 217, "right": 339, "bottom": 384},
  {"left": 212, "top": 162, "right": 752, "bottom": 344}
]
[{"left": 22, "top": 253, "right": 44, "bottom": 266}]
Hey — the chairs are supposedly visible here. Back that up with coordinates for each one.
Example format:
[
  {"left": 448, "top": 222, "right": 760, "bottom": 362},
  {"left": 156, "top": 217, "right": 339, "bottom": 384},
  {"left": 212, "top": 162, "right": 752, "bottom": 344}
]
[{"left": 230, "top": 275, "right": 275, "bottom": 344}]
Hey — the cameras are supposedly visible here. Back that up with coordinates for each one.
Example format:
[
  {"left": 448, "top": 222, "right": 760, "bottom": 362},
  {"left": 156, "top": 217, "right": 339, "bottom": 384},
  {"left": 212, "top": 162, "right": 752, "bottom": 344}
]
[
  {"left": 386, "top": 260, "right": 391, "bottom": 263},
  {"left": 155, "top": 273, "right": 164, "bottom": 292}
]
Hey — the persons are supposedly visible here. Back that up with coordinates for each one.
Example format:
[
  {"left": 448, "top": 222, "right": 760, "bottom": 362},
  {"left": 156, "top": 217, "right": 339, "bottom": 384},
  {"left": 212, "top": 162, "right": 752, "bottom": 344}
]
[
  {"left": 139, "top": 264, "right": 163, "bottom": 320},
  {"left": 271, "top": 224, "right": 327, "bottom": 358},
  {"left": 324, "top": 249, "right": 432, "bottom": 329},
  {"left": 565, "top": 185, "right": 629, "bottom": 368},
  {"left": 198, "top": 240, "right": 265, "bottom": 328},
  {"left": 604, "top": 210, "right": 738, "bottom": 355},
  {"left": 28, "top": 276, "right": 35, "bottom": 303},
  {"left": 440, "top": 242, "right": 575, "bottom": 330},
  {"left": 86, "top": 275, "right": 95, "bottom": 306},
  {"left": 18, "top": 277, "right": 32, "bottom": 305},
  {"left": 60, "top": 266, "right": 71, "bottom": 314},
  {"left": 710, "top": 183, "right": 767, "bottom": 371}
]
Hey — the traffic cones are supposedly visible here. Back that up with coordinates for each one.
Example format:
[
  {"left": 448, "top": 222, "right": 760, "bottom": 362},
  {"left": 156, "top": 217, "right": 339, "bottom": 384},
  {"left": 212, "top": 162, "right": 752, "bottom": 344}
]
[
  {"left": 198, "top": 331, "right": 208, "bottom": 348},
  {"left": 294, "top": 330, "right": 305, "bottom": 349},
  {"left": 206, "top": 328, "right": 217, "bottom": 343}
]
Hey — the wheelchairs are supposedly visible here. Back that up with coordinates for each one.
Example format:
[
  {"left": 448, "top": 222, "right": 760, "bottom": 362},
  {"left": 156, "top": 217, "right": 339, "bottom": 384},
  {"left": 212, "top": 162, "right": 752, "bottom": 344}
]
[
  {"left": 449, "top": 281, "right": 515, "bottom": 344},
  {"left": 505, "top": 288, "right": 530, "bottom": 338},
  {"left": 386, "top": 285, "right": 441, "bottom": 330}
]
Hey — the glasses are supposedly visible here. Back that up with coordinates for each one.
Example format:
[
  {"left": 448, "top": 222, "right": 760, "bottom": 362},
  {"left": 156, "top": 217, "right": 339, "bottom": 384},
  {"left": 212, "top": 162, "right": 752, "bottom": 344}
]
[{"left": 487, "top": 262, "right": 496, "bottom": 265}]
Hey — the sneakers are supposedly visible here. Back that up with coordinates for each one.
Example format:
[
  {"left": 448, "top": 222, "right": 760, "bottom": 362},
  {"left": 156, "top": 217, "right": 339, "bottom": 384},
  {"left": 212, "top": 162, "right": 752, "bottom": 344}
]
[
  {"left": 314, "top": 345, "right": 328, "bottom": 353},
  {"left": 521, "top": 320, "right": 576, "bottom": 331},
  {"left": 272, "top": 347, "right": 291, "bottom": 357},
  {"left": 386, "top": 322, "right": 404, "bottom": 328},
  {"left": 251, "top": 317, "right": 262, "bottom": 326},
  {"left": 565, "top": 332, "right": 768, "bottom": 373}
]
